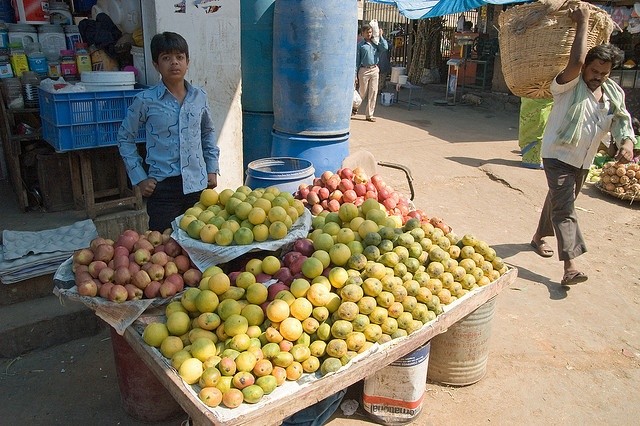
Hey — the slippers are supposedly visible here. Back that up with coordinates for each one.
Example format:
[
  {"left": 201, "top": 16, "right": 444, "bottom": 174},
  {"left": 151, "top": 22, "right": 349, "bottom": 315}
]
[
  {"left": 561, "top": 270, "right": 588, "bottom": 285},
  {"left": 530, "top": 240, "right": 554, "bottom": 257}
]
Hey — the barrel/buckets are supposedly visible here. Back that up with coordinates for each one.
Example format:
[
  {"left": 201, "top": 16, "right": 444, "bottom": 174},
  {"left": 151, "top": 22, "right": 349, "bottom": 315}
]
[
  {"left": 39, "top": 26, "right": 67, "bottom": 56},
  {"left": 11, "top": 0, "right": 50, "bottom": 25},
  {"left": 51, "top": 3, "right": 73, "bottom": 27},
  {"left": 111, "top": 324, "right": 183, "bottom": 423},
  {"left": 29, "top": 50, "right": 48, "bottom": 76},
  {"left": 14, "top": 50, "right": 30, "bottom": 78},
  {"left": 428, "top": 294, "right": 498, "bottom": 387},
  {"left": 245, "top": 157, "right": 315, "bottom": 196},
  {"left": 77, "top": 51, "right": 92, "bottom": 74},
  {"left": 0, "top": 20, "right": 9, "bottom": 48},
  {"left": 67, "top": 27, "right": 84, "bottom": 51},
  {"left": 272, "top": 0, "right": 359, "bottom": 137},
  {"left": 0, "top": 49, "right": 14, "bottom": 79},
  {"left": 242, "top": 112, "right": 274, "bottom": 186},
  {"left": 60, "top": 51, "right": 76, "bottom": 76},
  {"left": 381, "top": 92, "right": 395, "bottom": 106},
  {"left": 23, "top": 70, "right": 42, "bottom": 109},
  {"left": 363, "top": 342, "right": 431, "bottom": 425},
  {"left": 36, "top": 152, "right": 75, "bottom": 212},
  {"left": 239, "top": 0, "right": 273, "bottom": 112},
  {"left": 9, "top": 23, "right": 38, "bottom": 49},
  {"left": 130, "top": 45, "right": 146, "bottom": 84},
  {"left": 4, "top": 77, "right": 25, "bottom": 110},
  {"left": 91, "top": 1, "right": 108, "bottom": 21},
  {"left": 271, "top": 130, "right": 351, "bottom": 178},
  {"left": 108, "top": 1, "right": 141, "bottom": 34},
  {"left": 48, "top": 52, "right": 62, "bottom": 77},
  {"left": 80, "top": 71, "right": 136, "bottom": 92}
]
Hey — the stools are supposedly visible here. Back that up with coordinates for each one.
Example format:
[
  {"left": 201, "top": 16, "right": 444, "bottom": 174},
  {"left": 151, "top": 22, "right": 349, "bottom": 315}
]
[{"left": 69, "top": 147, "right": 142, "bottom": 220}]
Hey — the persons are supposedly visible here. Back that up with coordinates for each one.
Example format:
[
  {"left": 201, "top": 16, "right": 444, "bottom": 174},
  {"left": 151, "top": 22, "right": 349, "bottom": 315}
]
[
  {"left": 530, "top": 3, "right": 637, "bottom": 286},
  {"left": 351, "top": 25, "right": 389, "bottom": 123},
  {"left": 465, "top": 21, "right": 473, "bottom": 30},
  {"left": 115, "top": 31, "right": 221, "bottom": 228}
]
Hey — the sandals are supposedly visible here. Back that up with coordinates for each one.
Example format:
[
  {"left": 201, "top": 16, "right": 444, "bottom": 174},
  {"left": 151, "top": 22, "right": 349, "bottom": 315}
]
[
  {"left": 366, "top": 117, "right": 376, "bottom": 122},
  {"left": 352, "top": 108, "right": 358, "bottom": 115}
]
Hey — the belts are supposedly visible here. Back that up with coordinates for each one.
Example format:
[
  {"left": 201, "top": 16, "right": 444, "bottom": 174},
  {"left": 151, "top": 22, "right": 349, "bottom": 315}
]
[{"left": 360, "top": 65, "right": 376, "bottom": 68}]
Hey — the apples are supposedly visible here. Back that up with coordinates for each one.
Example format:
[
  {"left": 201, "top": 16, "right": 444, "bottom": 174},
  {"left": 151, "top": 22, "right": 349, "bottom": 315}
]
[
  {"left": 293, "top": 168, "right": 416, "bottom": 214},
  {"left": 179, "top": 187, "right": 302, "bottom": 242},
  {"left": 72, "top": 229, "right": 201, "bottom": 301},
  {"left": 307, "top": 210, "right": 510, "bottom": 377},
  {"left": 139, "top": 240, "right": 320, "bottom": 407}
]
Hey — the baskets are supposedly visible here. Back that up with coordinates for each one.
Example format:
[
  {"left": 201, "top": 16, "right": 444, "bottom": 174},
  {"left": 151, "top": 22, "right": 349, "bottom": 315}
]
[
  {"left": 594, "top": 182, "right": 639, "bottom": 205},
  {"left": 498, "top": 1, "right": 623, "bottom": 98}
]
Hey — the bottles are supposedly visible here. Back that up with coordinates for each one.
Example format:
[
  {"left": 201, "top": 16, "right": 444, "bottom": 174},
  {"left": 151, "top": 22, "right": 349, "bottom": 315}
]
[
  {"left": 9, "top": 41, "right": 30, "bottom": 77},
  {"left": 47, "top": 44, "right": 62, "bottom": 79},
  {"left": 60, "top": 49, "right": 78, "bottom": 80},
  {"left": 28, "top": 42, "right": 48, "bottom": 74},
  {"left": 74, "top": 42, "right": 93, "bottom": 75}
]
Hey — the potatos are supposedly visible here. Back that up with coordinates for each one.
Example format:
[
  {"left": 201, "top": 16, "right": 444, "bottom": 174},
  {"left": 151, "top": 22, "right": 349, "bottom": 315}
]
[{"left": 597, "top": 161, "right": 640, "bottom": 197}]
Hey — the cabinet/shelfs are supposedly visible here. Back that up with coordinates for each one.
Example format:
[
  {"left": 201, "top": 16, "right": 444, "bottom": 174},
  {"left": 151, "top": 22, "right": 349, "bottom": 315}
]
[{"left": 450, "top": 33, "right": 489, "bottom": 84}]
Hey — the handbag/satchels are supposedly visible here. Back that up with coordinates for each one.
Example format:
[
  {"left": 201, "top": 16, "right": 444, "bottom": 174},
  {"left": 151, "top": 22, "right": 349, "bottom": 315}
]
[{"left": 369, "top": 19, "right": 380, "bottom": 46}]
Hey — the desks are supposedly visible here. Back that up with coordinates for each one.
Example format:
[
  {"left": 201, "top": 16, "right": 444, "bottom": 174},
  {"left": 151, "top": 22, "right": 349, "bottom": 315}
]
[{"left": 387, "top": 81, "right": 423, "bottom": 111}]
